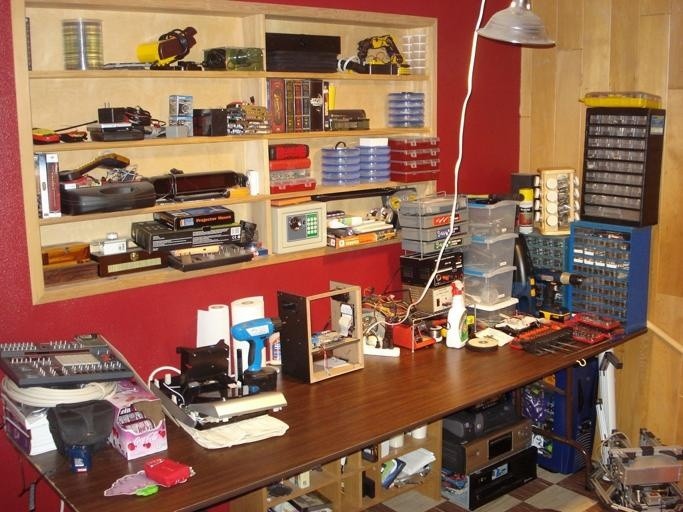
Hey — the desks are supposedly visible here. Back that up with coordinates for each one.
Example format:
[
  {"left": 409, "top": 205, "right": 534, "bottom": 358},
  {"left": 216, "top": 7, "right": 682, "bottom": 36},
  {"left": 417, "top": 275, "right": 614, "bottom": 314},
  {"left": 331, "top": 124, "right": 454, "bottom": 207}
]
[{"left": 3, "top": 324, "right": 649, "bottom": 512}]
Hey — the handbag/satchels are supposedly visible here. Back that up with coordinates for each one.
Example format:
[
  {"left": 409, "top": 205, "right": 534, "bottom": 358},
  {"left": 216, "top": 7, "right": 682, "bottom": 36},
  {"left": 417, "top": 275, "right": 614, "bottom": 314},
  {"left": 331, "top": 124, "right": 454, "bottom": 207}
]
[{"left": 47, "top": 399, "right": 119, "bottom": 457}]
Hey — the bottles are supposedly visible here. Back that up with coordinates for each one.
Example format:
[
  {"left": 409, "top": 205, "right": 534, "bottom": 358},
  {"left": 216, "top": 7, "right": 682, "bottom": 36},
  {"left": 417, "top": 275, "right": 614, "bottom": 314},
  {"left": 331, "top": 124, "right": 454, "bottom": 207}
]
[{"left": 518, "top": 188, "right": 534, "bottom": 235}]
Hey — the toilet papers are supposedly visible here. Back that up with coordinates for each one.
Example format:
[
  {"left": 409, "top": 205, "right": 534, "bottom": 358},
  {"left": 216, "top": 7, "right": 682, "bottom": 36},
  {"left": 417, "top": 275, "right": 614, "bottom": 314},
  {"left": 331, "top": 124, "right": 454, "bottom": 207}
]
[
  {"left": 196, "top": 302, "right": 229, "bottom": 347},
  {"left": 231, "top": 294, "right": 265, "bottom": 372}
]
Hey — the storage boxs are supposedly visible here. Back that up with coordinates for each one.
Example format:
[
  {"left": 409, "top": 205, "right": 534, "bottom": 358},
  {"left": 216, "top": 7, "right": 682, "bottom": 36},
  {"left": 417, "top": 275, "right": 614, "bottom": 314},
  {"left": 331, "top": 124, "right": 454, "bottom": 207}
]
[{"left": 465, "top": 197, "right": 520, "bottom": 327}]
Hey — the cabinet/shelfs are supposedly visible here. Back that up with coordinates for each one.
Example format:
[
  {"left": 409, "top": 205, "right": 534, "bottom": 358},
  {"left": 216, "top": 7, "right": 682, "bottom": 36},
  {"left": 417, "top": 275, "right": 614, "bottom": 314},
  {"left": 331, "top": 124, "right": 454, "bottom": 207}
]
[
  {"left": 229, "top": 417, "right": 443, "bottom": 512},
  {"left": 10, "top": 1, "right": 441, "bottom": 305}
]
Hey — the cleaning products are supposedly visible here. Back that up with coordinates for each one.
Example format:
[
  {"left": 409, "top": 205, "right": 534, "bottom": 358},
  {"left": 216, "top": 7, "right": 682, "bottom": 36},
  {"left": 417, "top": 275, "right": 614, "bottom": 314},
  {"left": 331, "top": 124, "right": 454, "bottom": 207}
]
[{"left": 447, "top": 278, "right": 469, "bottom": 349}]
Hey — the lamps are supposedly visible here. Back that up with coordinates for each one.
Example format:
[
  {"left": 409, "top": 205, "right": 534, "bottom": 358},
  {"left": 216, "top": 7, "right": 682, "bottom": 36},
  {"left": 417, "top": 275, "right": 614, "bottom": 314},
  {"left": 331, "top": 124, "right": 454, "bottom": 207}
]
[{"left": 476, "top": 1, "right": 557, "bottom": 47}]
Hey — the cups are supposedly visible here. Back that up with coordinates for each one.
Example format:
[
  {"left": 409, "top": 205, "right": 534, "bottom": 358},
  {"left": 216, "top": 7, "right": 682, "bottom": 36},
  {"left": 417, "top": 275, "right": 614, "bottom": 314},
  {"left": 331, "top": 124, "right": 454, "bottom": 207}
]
[
  {"left": 410, "top": 424, "right": 427, "bottom": 441},
  {"left": 389, "top": 432, "right": 405, "bottom": 448}
]
